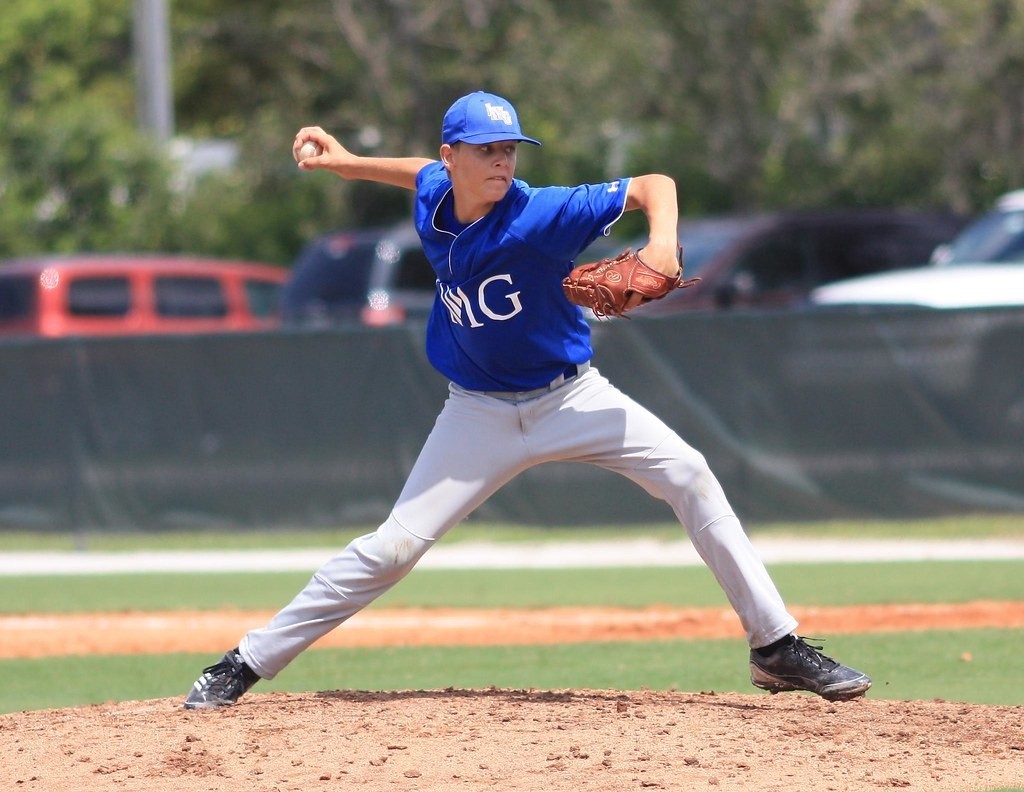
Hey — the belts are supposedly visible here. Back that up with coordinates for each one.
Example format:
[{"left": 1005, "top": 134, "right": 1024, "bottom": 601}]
[{"left": 563, "top": 364, "right": 577, "bottom": 381}]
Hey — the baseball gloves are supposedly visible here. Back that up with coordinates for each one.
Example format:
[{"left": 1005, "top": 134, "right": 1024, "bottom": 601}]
[{"left": 562, "top": 244, "right": 684, "bottom": 318}]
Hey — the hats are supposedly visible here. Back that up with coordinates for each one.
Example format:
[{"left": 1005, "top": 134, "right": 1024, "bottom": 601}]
[{"left": 442, "top": 90, "right": 542, "bottom": 145}]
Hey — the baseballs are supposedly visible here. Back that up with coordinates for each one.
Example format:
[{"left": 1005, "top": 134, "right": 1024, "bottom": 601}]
[{"left": 298, "top": 140, "right": 323, "bottom": 162}]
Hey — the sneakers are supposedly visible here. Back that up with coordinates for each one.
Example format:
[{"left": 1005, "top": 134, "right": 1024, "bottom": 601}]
[
  {"left": 184, "top": 650, "right": 262, "bottom": 710},
  {"left": 749, "top": 635, "right": 871, "bottom": 698}
]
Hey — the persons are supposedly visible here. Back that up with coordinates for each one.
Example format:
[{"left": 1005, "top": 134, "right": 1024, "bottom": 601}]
[{"left": 181, "top": 89, "right": 872, "bottom": 709}]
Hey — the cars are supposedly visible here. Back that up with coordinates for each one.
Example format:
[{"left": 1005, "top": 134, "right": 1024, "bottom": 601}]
[
  {"left": 275, "top": 227, "right": 438, "bottom": 327},
  {"left": 0, "top": 259, "right": 407, "bottom": 336},
  {"left": 592, "top": 208, "right": 968, "bottom": 319},
  {"left": 790, "top": 188, "right": 1023, "bottom": 310}
]
